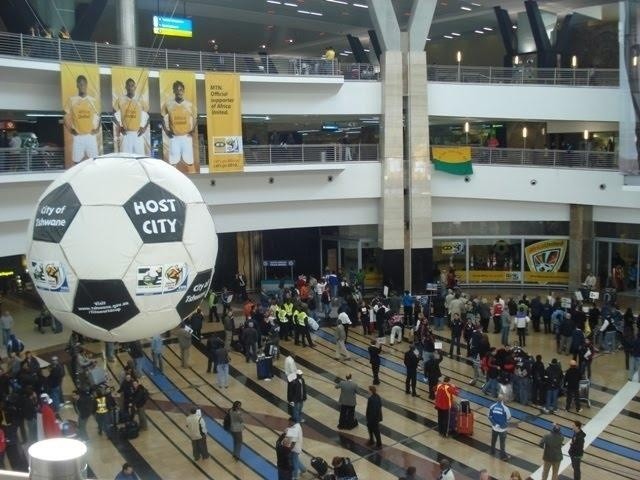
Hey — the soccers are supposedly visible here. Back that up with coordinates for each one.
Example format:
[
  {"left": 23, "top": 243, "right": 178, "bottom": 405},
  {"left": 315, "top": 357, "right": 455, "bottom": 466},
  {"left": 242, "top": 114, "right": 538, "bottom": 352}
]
[{"left": 23, "top": 150, "right": 221, "bottom": 345}]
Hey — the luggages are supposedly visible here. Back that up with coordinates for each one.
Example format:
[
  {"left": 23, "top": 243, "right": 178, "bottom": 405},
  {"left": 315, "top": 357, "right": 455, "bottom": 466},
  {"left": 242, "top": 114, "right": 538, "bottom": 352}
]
[
  {"left": 311, "top": 456, "right": 328, "bottom": 476},
  {"left": 455, "top": 404, "right": 473, "bottom": 436},
  {"left": 307, "top": 317, "right": 319, "bottom": 331},
  {"left": 256, "top": 355, "right": 274, "bottom": 379},
  {"left": 497, "top": 382, "right": 513, "bottom": 403},
  {"left": 112, "top": 424, "right": 126, "bottom": 444},
  {"left": 107, "top": 423, "right": 116, "bottom": 440},
  {"left": 87, "top": 367, "right": 105, "bottom": 386},
  {"left": 126, "top": 420, "right": 138, "bottom": 438},
  {"left": 450, "top": 404, "right": 461, "bottom": 432}
]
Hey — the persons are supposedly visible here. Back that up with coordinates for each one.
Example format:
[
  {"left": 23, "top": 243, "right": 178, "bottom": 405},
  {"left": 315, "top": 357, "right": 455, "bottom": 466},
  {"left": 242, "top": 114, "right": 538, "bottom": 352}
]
[
  {"left": 366, "top": 385, "right": 382, "bottom": 450},
  {"left": 287, "top": 369, "right": 307, "bottom": 423},
  {"left": 335, "top": 372, "right": 360, "bottom": 426},
  {"left": 510, "top": 470, "right": 522, "bottom": 480},
  {"left": 331, "top": 456, "right": 358, "bottom": 480},
  {"left": 178, "top": 324, "right": 191, "bottom": 369},
  {"left": 445, "top": 264, "right": 532, "bottom": 401},
  {"left": 437, "top": 458, "right": 455, "bottom": 480},
  {"left": 404, "top": 344, "right": 421, "bottom": 397},
  {"left": 568, "top": 420, "right": 587, "bottom": 480},
  {"left": 540, "top": 422, "right": 564, "bottom": 480},
  {"left": 0, "top": 300, "right": 148, "bottom": 463},
  {"left": 58, "top": 25, "right": 69, "bottom": 39},
  {"left": 275, "top": 428, "right": 298, "bottom": 480},
  {"left": 542, "top": 137, "right": 614, "bottom": 166},
  {"left": 533, "top": 270, "right": 640, "bottom": 368},
  {"left": 487, "top": 135, "right": 499, "bottom": 162},
  {"left": 436, "top": 375, "right": 458, "bottom": 437},
  {"left": 284, "top": 349, "right": 298, "bottom": 408},
  {"left": 565, "top": 361, "right": 583, "bottom": 413},
  {"left": 208, "top": 288, "right": 220, "bottom": 323},
  {"left": 214, "top": 341, "right": 231, "bottom": 388},
  {"left": 206, "top": 332, "right": 223, "bottom": 373},
  {"left": 115, "top": 463, "right": 141, "bottom": 480},
  {"left": 398, "top": 466, "right": 419, "bottom": 480},
  {"left": 161, "top": 80, "right": 197, "bottom": 173},
  {"left": 282, "top": 417, "right": 306, "bottom": 477},
  {"left": 224, "top": 312, "right": 233, "bottom": 351},
  {"left": 344, "top": 134, "right": 352, "bottom": 160},
  {"left": 543, "top": 358, "right": 564, "bottom": 413},
  {"left": 531, "top": 355, "right": 545, "bottom": 405},
  {"left": 250, "top": 135, "right": 262, "bottom": 162},
  {"left": 239, "top": 267, "right": 433, "bottom": 347},
  {"left": 479, "top": 469, "right": 498, "bottom": 480},
  {"left": 63, "top": 75, "right": 100, "bottom": 166},
  {"left": 209, "top": 44, "right": 219, "bottom": 71},
  {"left": 424, "top": 347, "right": 444, "bottom": 400},
  {"left": 8, "top": 131, "right": 22, "bottom": 171},
  {"left": 113, "top": 78, "right": 150, "bottom": 157},
  {"left": 185, "top": 407, "right": 210, "bottom": 461},
  {"left": 281, "top": 427, "right": 290, "bottom": 433},
  {"left": 151, "top": 334, "right": 163, "bottom": 376},
  {"left": 488, "top": 394, "right": 512, "bottom": 461},
  {"left": 29, "top": 22, "right": 40, "bottom": 38},
  {"left": 325, "top": 46, "right": 335, "bottom": 61},
  {"left": 221, "top": 286, "right": 234, "bottom": 315},
  {"left": 368, "top": 340, "right": 383, "bottom": 385},
  {"left": 191, "top": 307, "right": 203, "bottom": 341},
  {"left": 222, "top": 401, "right": 245, "bottom": 463}
]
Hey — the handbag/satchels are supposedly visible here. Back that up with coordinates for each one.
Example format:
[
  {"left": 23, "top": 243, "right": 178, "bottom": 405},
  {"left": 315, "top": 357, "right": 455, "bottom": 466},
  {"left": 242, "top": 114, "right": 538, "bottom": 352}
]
[
  {"left": 224, "top": 409, "right": 231, "bottom": 430},
  {"left": 344, "top": 418, "right": 358, "bottom": 430},
  {"left": 198, "top": 417, "right": 206, "bottom": 440}
]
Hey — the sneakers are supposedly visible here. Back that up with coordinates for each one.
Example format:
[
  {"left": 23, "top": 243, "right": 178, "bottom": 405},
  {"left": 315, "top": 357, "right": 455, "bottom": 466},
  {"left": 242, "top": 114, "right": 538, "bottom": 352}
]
[
  {"left": 543, "top": 407, "right": 549, "bottom": 413},
  {"left": 579, "top": 408, "right": 583, "bottom": 413},
  {"left": 563, "top": 407, "right": 568, "bottom": 411},
  {"left": 491, "top": 450, "right": 496, "bottom": 454},
  {"left": 554, "top": 409, "right": 560, "bottom": 413},
  {"left": 299, "top": 419, "right": 305, "bottom": 422},
  {"left": 443, "top": 433, "right": 449, "bottom": 437},
  {"left": 503, "top": 455, "right": 511, "bottom": 461},
  {"left": 439, "top": 430, "right": 442, "bottom": 434}
]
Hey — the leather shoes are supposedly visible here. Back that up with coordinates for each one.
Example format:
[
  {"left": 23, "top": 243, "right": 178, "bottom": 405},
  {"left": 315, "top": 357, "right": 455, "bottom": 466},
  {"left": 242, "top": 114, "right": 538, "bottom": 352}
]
[
  {"left": 194, "top": 456, "right": 201, "bottom": 461},
  {"left": 412, "top": 394, "right": 420, "bottom": 397},
  {"left": 406, "top": 391, "right": 411, "bottom": 394},
  {"left": 390, "top": 343, "right": 395, "bottom": 346},
  {"left": 365, "top": 441, "right": 375, "bottom": 445},
  {"left": 344, "top": 358, "right": 351, "bottom": 360},
  {"left": 375, "top": 381, "right": 381, "bottom": 385},
  {"left": 484, "top": 391, "right": 489, "bottom": 394},
  {"left": 372, "top": 445, "right": 382, "bottom": 450},
  {"left": 203, "top": 454, "right": 210, "bottom": 458},
  {"left": 337, "top": 424, "right": 343, "bottom": 430},
  {"left": 334, "top": 357, "right": 339, "bottom": 360},
  {"left": 494, "top": 395, "right": 498, "bottom": 398},
  {"left": 397, "top": 341, "right": 402, "bottom": 343}
]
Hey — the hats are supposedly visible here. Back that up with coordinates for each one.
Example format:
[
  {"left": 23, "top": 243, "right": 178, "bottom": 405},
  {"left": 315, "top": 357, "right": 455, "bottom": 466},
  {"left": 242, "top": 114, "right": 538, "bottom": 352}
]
[
  {"left": 569, "top": 360, "right": 576, "bottom": 366},
  {"left": 553, "top": 422, "right": 561, "bottom": 431},
  {"left": 296, "top": 370, "right": 304, "bottom": 374}
]
